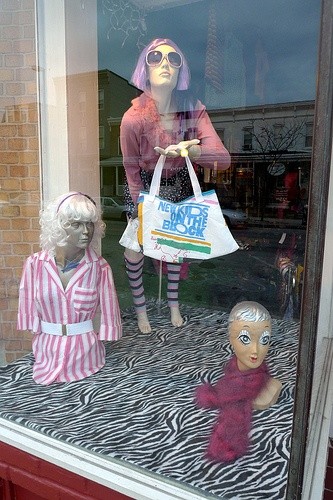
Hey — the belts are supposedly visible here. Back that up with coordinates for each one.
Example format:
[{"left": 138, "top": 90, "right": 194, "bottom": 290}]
[{"left": 39, "top": 320, "right": 93, "bottom": 336}]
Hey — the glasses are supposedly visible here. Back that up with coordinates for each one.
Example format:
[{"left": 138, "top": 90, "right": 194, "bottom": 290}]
[{"left": 145, "top": 49, "right": 182, "bottom": 69}]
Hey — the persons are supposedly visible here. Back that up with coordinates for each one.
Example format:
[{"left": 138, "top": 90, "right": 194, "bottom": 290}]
[
  {"left": 195, "top": 301, "right": 281, "bottom": 458},
  {"left": 119, "top": 38, "right": 231, "bottom": 334},
  {"left": 17, "top": 193, "right": 121, "bottom": 384}
]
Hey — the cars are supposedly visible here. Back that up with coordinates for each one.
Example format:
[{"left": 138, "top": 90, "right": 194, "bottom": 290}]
[
  {"left": 89, "top": 195, "right": 133, "bottom": 221},
  {"left": 221, "top": 208, "right": 249, "bottom": 229}
]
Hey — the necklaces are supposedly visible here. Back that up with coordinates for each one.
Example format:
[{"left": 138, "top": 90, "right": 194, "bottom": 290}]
[{"left": 60, "top": 251, "right": 83, "bottom": 272}]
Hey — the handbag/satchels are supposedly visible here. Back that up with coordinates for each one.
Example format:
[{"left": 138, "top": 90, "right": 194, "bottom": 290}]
[{"left": 118, "top": 145, "right": 240, "bottom": 263}]
